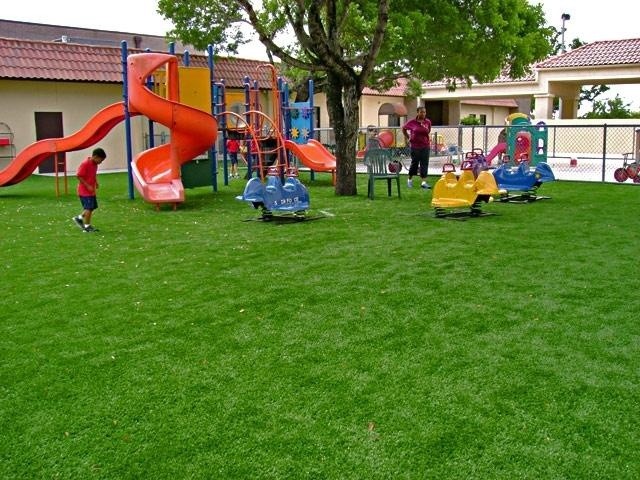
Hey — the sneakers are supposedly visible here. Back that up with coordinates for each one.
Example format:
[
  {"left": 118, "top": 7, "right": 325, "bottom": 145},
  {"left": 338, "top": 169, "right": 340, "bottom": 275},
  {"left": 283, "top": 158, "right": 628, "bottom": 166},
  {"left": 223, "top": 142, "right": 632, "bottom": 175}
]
[
  {"left": 83, "top": 225, "right": 99, "bottom": 233},
  {"left": 407, "top": 179, "right": 413, "bottom": 188},
  {"left": 421, "top": 184, "right": 431, "bottom": 189},
  {"left": 234, "top": 174, "right": 241, "bottom": 179},
  {"left": 72, "top": 215, "right": 84, "bottom": 230},
  {"left": 228, "top": 175, "right": 235, "bottom": 179}
]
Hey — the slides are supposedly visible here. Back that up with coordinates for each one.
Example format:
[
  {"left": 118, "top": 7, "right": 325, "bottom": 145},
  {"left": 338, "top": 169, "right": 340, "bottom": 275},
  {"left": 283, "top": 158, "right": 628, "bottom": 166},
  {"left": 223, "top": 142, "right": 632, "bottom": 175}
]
[
  {"left": 0, "top": 101, "right": 142, "bottom": 188},
  {"left": 285, "top": 138, "right": 337, "bottom": 172},
  {"left": 486, "top": 143, "right": 506, "bottom": 161},
  {"left": 131, "top": 86, "right": 218, "bottom": 203}
]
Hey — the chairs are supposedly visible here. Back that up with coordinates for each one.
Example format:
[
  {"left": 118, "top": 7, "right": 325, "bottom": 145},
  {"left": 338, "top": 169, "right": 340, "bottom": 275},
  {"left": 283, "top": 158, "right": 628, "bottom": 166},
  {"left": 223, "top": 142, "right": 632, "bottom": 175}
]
[{"left": 363, "top": 147, "right": 401, "bottom": 200}]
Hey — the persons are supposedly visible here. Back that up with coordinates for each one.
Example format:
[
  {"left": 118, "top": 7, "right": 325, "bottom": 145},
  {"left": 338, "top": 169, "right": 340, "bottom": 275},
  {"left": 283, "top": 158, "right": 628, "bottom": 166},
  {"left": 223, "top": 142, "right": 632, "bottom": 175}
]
[
  {"left": 226, "top": 139, "right": 242, "bottom": 178},
  {"left": 499, "top": 154, "right": 512, "bottom": 172},
  {"left": 71, "top": 147, "right": 107, "bottom": 234},
  {"left": 401, "top": 106, "right": 433, "bottom": 190}
]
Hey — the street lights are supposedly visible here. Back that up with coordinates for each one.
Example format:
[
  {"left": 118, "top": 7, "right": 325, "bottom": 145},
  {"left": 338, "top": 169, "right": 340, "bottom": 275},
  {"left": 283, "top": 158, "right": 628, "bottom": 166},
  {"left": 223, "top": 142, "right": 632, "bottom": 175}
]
[{"left": 560, "top": 12, "right": 571, "bottom": 55}]
